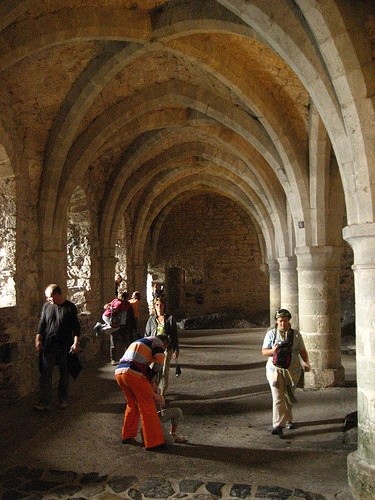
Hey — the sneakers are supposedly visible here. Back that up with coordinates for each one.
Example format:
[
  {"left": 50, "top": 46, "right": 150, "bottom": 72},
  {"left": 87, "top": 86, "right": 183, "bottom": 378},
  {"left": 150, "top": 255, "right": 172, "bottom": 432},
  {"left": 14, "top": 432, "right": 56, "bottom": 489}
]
[
  {"left": 32, "top": 402, "right": 52, "bottom": 411},
  {"left": 57, "top": 400, "right": 68, "bottom": 409},
  {"left": 285, "top": 420, "right": 295, "bottom": 430},
  {"left": 169, "top": 433, "right": 189, "bottom": 442},
  {"left": 271, "top": 426, "right": 283, "bottom": 434}
]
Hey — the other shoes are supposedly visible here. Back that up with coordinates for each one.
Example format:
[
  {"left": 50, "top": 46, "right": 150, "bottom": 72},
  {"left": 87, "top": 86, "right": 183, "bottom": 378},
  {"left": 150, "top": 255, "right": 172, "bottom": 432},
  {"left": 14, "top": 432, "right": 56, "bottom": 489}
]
[{"left": 111, "top": 360, "right": 116, "bottom": 366}]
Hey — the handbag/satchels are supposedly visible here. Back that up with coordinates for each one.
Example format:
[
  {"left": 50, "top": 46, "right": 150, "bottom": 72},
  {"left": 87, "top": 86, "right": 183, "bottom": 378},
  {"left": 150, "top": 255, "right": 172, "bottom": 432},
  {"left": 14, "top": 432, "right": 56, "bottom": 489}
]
[
  {"left": 176, "top": 365, "right": 181, "bottom": 374},
  {"left": 273, "top": 329, "right": 294, "bottom": 369}
]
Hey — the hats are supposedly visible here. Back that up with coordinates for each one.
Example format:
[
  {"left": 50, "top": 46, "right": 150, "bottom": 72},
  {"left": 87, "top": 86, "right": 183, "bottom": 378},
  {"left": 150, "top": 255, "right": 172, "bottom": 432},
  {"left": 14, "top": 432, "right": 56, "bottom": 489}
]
[{"left": 274, "top": 309, "right": 292, "bottom": 319}]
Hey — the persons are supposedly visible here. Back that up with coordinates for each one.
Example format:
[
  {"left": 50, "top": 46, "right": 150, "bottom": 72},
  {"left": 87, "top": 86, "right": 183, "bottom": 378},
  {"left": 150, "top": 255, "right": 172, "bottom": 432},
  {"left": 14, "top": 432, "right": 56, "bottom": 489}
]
[
  {"left": 262, "top": 309, "right": 313, "bottom": 437},
  {"left": 144, "top": 297, "right": 180, "bottom": 404},
  {"left": 114, "top": 333, "right": 186, "bottom": 451},
  {"left": 93, "top": 274, "right": 141, "bottom": 365},
  {"left": 35, "top": 284, "right": 80, "bottom": 412}
]
